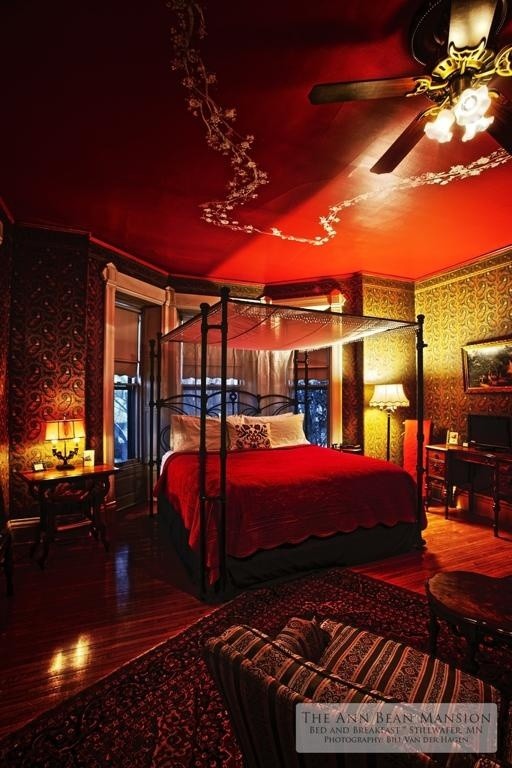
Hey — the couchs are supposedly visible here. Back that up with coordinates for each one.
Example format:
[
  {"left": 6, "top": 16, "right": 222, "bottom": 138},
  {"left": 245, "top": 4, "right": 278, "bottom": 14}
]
[{"left": 203, "top": 611, "right": 511, "bottom": 767}]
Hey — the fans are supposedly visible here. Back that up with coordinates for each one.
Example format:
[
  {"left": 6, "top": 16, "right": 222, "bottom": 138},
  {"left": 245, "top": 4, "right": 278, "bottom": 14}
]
[{"left": 308, "top": 0, "right": 512, "bottom": 176}]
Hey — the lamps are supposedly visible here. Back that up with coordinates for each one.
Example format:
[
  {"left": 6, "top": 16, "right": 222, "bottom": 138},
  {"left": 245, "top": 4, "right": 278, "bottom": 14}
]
[
  {"left": 45, "top": 413, "right": 89, "bottom": 471},
  {"left": 408, "top": 67, "right": 510, "bottom": 146},
  {"left": 369, "top": 383, "right": 411, "bottom": 460}
]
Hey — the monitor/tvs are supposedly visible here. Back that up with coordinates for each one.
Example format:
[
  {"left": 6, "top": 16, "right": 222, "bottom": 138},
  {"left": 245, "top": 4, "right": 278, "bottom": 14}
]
[{"left": 467, "top": 414, "right": 512, "bottom": 451}]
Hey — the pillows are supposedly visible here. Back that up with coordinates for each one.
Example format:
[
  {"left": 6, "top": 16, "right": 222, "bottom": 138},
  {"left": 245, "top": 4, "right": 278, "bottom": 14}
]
[{"left": 168, "top": 412, "right": 312, "bottom": 454}]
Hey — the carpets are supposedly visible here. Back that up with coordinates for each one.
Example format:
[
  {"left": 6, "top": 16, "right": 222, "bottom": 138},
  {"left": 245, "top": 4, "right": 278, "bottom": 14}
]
[{"left": 2, "top": 570, "right": 510, "bottom": 766}]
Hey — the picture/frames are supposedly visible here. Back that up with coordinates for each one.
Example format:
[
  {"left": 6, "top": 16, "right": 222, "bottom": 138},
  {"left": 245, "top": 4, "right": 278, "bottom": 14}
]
[{"left": 459, "top": 334, "right": 511, "bottom": 395}]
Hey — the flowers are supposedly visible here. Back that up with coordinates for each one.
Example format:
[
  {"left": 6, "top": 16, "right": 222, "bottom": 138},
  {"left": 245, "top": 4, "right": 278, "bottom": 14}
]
[
  {"left": 271, "top": 147, "right": 511, "bottom": 246},
  {"left": 159, "top": 1, "right": 270, "bottom": 232}
]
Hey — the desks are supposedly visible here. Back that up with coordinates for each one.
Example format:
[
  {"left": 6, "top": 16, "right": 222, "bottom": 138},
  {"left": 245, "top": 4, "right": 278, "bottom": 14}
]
[
  {"left": 423, "top": 442, "right": 511, "bottom": 537},
  {"left": 18, "top": 464, "right": 121, "bottom": 559}
]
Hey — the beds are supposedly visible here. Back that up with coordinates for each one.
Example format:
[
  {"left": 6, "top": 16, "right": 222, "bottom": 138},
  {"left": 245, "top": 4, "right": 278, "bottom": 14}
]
[{"left": 148, "top": 283, "right": 429, "bottom": 607}]
